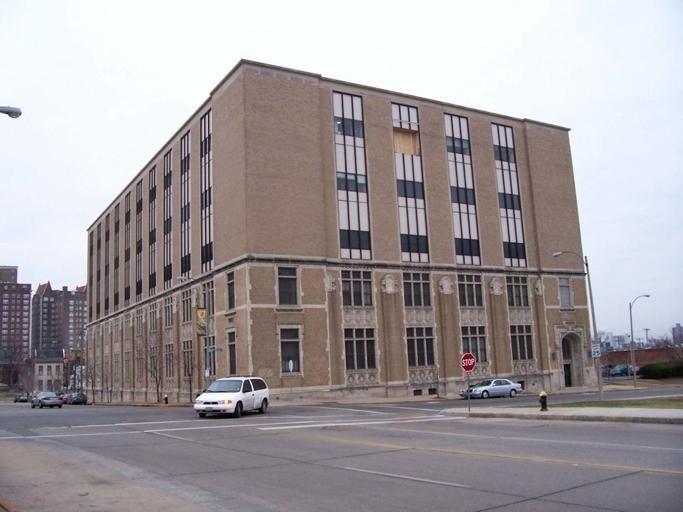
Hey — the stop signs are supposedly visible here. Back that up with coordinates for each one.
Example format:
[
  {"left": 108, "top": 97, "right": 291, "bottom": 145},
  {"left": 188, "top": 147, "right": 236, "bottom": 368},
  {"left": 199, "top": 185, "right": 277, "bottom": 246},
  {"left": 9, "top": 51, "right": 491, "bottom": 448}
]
[{"left": 460, "top": 353, "right": 476, "bottom": 372}]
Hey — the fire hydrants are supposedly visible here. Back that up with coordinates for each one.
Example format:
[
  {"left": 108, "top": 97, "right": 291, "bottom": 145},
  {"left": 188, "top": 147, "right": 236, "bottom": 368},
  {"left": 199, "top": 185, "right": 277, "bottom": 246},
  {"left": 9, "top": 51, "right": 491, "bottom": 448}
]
[
  {"left": 539, "top": 392, "right": 548, "bottom": 411},
  {"left": 163, "top": 395, "right": 168, "bottom": 404}
]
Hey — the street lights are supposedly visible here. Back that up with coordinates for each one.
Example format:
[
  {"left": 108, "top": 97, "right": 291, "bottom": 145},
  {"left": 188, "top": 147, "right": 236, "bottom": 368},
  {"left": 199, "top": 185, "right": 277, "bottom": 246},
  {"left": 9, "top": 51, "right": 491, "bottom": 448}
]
[
  {"left": 0, "top": 106, "right": 22, "bottom": 119},
  {"left": 552, "top": 250, "right": 604, "bottom": 401},
  {"left": 629, "top": 295, "right": 650, "bottom": 389}
]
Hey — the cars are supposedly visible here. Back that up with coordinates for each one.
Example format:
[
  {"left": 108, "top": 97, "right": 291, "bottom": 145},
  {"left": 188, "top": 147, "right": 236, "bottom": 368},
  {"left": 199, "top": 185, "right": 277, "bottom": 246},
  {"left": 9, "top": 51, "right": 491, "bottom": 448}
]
[
  {"left": 175, "top": 276, "right": 210, "bottom": 389},
  {"left": 193, "top": 376, "right": 270, "bottom": 418},
  {"left": 601, "top": 364, "right": 640, "bottom": 376},
  {"left": 463, "top": 378, "right": 522, "bottom": 399},
  {"left": 14, "top": 391, "right": 86, "bottom": 408}
]
